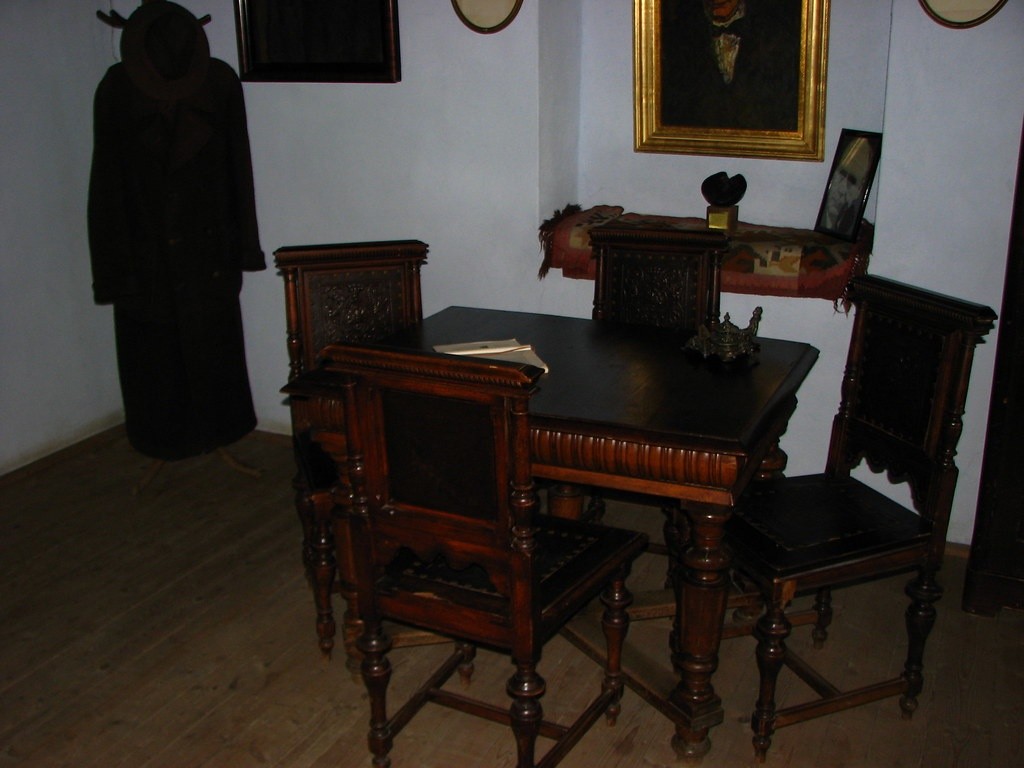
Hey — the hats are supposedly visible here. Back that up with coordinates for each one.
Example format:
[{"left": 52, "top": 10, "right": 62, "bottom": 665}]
[{"left": 119, "top": 1, "right": 210, "bottom": 101}]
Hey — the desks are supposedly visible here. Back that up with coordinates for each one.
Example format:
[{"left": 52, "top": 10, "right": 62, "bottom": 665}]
[
  {"left": 279, "top": 300, "right": 819, "bottom": 766},
  {"left": 539, "top": 206, "right": 878, "bottom": 310}
]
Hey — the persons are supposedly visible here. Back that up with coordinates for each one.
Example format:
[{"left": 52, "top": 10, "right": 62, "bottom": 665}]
[
  {"left": 667, "top": 0, "right": 792, "bottom": 131},
  {"left": 821, "top": 136, "right": 874, "bottom": 236}
]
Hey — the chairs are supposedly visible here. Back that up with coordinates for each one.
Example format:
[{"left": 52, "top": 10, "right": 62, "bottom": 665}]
[
  {"left": 273, "top": 240, "right": 430, "bottom": 591},
  {"left": 314, "top": 343, "right": 650, "bottom": 768},
  {"left": 666, "top": 274, "right": 996, "bottom": 763},
  {"left": 580, "top": 226, "right": 728, "bottom": 584}
]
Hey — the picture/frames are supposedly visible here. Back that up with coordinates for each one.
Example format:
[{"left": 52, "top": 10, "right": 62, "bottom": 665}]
[
  {"left": 814, "top": 128, "right": 883, "bottom": 241},
  {"left": 451, "top": 0, "right": 523, "bottom": 34},
  {"left": 918, "top": 0, "right": 1007, "bottom": 29},
  {"left": 234, "top": 0, "right": 402, "bottom": 85},
  {"left": 635, "top": 1, "right": 832, "bottom": 161}
]
